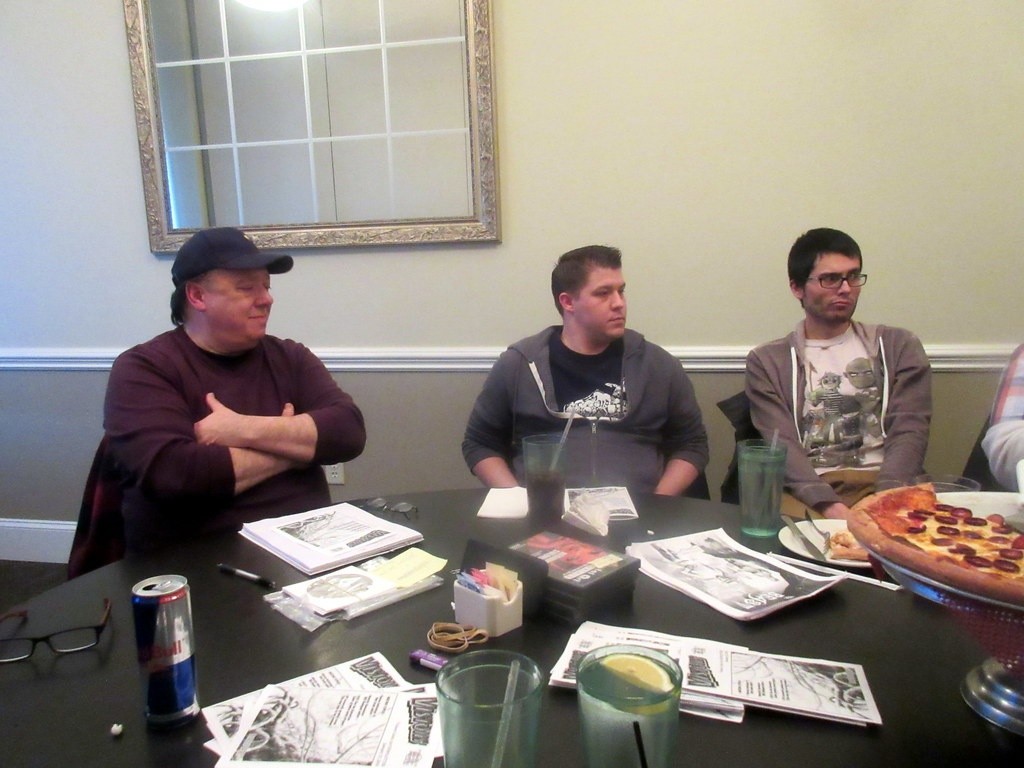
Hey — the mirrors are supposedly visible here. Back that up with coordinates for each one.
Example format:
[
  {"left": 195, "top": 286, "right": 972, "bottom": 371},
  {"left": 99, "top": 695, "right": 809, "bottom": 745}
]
[{"left": 122, "top": 1, "right": 504, "bottom": 258}]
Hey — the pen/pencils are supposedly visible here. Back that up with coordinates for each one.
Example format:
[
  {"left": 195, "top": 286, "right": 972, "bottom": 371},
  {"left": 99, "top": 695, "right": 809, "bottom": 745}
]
[{"left": 217, "top": 560, "right": 278, "bottom": 592}]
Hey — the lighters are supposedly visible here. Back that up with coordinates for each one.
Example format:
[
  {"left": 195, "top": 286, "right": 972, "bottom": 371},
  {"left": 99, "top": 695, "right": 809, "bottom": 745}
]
[{"left": 410, "top": 649, "right": 460, "bottom": 673}]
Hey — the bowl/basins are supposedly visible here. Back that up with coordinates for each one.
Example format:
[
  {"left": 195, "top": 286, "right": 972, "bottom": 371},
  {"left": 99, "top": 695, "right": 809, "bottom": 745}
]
[{"left": 851, "top": 492, "right": 1023, "bottom": 738}]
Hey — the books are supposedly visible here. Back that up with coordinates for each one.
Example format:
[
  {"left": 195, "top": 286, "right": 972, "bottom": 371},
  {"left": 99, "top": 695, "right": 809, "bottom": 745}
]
[
  {"left": 564, "top": 488, "right": 639, "bottom": 521},
  {"left": 547, "top": 620, "right": 882, "bottom": 726}
]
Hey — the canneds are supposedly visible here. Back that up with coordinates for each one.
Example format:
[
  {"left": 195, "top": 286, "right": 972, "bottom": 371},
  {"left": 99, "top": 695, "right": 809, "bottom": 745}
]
[{"left": 131, "top": 575, "right": 200, "bottom": 728}]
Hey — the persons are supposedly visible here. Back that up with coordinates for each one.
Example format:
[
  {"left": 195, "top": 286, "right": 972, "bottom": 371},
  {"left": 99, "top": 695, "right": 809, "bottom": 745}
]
[
  {"left": 745, "top": 227, "right": 932, "bottom": 520},
  {"left": 461, "top": 244, "right": 709, "bottom": 497},
  {"left": 102, "top": 226, "right": 367, "bottom": 554},
  {"left": 980, "top": 343, "right": 1024, "bottom": 492}
]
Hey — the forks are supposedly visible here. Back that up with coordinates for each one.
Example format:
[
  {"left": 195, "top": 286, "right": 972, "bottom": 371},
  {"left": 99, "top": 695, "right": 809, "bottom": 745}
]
[{"left": 804, "top": 509, "right": 831, "bottom": 554}]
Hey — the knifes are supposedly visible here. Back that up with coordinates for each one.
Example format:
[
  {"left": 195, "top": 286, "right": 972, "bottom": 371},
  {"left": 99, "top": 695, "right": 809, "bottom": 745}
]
[{"left": 781, "top": 515, "right": 827, "bottom": 561}]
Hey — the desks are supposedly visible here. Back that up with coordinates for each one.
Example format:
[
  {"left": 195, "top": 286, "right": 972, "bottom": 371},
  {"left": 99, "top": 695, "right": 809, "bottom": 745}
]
[{"left": 0, "top": 489, "right": 1023, "bottom": 768}]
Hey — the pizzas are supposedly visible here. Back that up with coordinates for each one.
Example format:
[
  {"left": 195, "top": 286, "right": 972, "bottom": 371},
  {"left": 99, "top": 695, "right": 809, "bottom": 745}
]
[
  {"left": 845, "top": 481, "right": 1024, "bottom": 607},
  {"left": 829, "top": 529, "right": 871, "bottom": 561}
]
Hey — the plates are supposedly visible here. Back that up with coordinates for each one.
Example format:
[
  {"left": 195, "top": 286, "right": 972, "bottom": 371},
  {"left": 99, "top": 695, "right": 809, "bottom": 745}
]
[{"left": 778, "top": 518, "right": 874, "bottom": 567}]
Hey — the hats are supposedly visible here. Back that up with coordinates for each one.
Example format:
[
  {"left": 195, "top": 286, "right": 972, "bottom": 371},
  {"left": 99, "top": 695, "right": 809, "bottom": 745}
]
[{"left": 171, "top": 227, "right": 299, "bottom": 276}]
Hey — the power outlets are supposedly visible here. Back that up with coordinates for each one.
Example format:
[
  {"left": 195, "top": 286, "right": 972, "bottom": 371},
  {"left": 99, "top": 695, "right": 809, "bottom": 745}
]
[{"left": 325, "top": 463, "right": 344, "bottom": 484}]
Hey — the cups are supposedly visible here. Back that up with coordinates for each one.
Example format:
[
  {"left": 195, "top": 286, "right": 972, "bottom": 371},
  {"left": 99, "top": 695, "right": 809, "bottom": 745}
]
[
  {"left": 522, "top": 435, "right": 569, "bottom": 528},
  {"left": 434, "top": 649, "right": 545, "bottom": 768},
  {"left": 916, "top": 473, "right": 981, "bottom": 494},
  {"left": 736, "top": 438, "right": 788, "bottom": 537},
  {"left": 575, "top": 645, "right": 683, "bottom": 768}
]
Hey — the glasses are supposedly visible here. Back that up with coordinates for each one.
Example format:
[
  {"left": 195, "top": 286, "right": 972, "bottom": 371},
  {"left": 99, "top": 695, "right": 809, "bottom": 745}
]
[
  {"left": 0, "top": 594, "right": 112, "bottom": 663},
  {"left": 802, "top": 273, "right": 868, "bottom": 291},
  {"left": 362, "top": 497, "right": 419, "bottom": 520}
]
